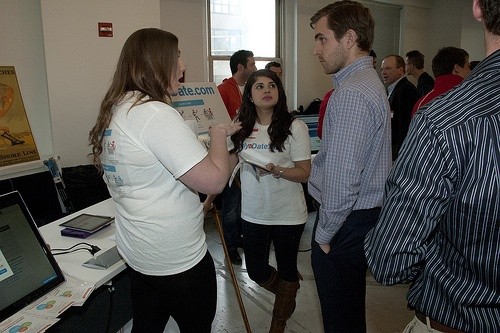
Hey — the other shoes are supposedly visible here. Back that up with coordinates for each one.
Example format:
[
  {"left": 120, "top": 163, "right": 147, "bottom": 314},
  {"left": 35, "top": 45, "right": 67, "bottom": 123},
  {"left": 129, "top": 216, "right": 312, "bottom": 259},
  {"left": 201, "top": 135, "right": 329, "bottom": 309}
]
[{"left": 227, "top": 246, "right": 242, "bottom": 265}]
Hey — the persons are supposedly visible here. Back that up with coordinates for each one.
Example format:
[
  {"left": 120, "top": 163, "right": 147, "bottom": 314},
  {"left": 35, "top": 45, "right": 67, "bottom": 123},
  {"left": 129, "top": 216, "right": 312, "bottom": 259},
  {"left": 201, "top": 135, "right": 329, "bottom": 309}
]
[
  {"left": 369, "top": 50, "right": 378, "bottom": 70},
  {"left": 88, "top": 27, "right": 242, "bottom": 333},
  {"left": 309, "top": 0, "right": 392, "bottom": 333},
  {"left": 202, "top": 69, "right": 311, "bottom": 333},
  {"left": 266, "top": 62, "right": 282, "bottom": 82},
  {"left": 380, "top": 54, "right": 419, "bottom": 163},
  {"left": 214, "top": 50, "right": 257, "bottom": 266},
  {"left": 405, "top": 50, "right": 434, "bottom": 96},
  {"left": 412, "top": 46, "right": 471, "bottom": 120},
  {"left": 364, "top": 0, "right": 500, "bottom": 333}
]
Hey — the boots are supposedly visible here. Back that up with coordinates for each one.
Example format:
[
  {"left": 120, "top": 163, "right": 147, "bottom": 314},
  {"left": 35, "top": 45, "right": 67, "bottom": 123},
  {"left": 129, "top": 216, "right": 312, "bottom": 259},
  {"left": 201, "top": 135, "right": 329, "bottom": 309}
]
[
  {"left": 270, "top": 270, "right": 303, "bottom": 333},
  {"left": 257, "top": 267, "right": 300, "bottom": 318}
]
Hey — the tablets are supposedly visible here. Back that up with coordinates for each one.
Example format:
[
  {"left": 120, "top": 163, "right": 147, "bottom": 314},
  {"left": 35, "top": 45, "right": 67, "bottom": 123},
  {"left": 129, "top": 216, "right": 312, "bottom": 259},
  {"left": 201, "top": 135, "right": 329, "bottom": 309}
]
[
  {"left": 58, "top": 213, "right": 115, "bottom": 234},
  {"left": 0, "top": 191, "right": 67, "bottom": 324}
]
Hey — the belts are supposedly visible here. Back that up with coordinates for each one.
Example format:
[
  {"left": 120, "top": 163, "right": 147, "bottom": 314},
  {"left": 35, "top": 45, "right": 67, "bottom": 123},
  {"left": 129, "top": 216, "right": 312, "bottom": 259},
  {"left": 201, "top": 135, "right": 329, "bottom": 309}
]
[{"left": 415, "top": 309, "right": 468, "bottom": 333}]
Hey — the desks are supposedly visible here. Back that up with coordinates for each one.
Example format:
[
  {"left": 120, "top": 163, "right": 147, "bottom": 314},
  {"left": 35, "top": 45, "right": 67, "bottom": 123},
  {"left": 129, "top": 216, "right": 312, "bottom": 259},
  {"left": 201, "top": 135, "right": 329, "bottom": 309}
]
[
  {"left": 0, "top": 198, "right": 252, "bottom": 333},
  {"left": 0, "top": 158, "right": 66, "bottom": 182}
]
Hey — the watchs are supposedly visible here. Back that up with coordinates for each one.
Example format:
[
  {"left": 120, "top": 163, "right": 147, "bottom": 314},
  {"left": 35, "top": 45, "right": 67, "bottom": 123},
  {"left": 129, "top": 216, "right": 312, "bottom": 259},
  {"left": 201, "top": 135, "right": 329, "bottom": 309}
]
[{"left": 272, "top": 167, "right": 283, "bottom": 180}]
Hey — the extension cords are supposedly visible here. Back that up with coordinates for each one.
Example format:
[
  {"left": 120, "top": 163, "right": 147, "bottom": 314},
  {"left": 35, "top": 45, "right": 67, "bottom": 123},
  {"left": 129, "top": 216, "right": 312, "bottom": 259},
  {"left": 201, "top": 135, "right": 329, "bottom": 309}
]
[{"left": 94, "top": 243, "right": 117, "bottom": 261}]
